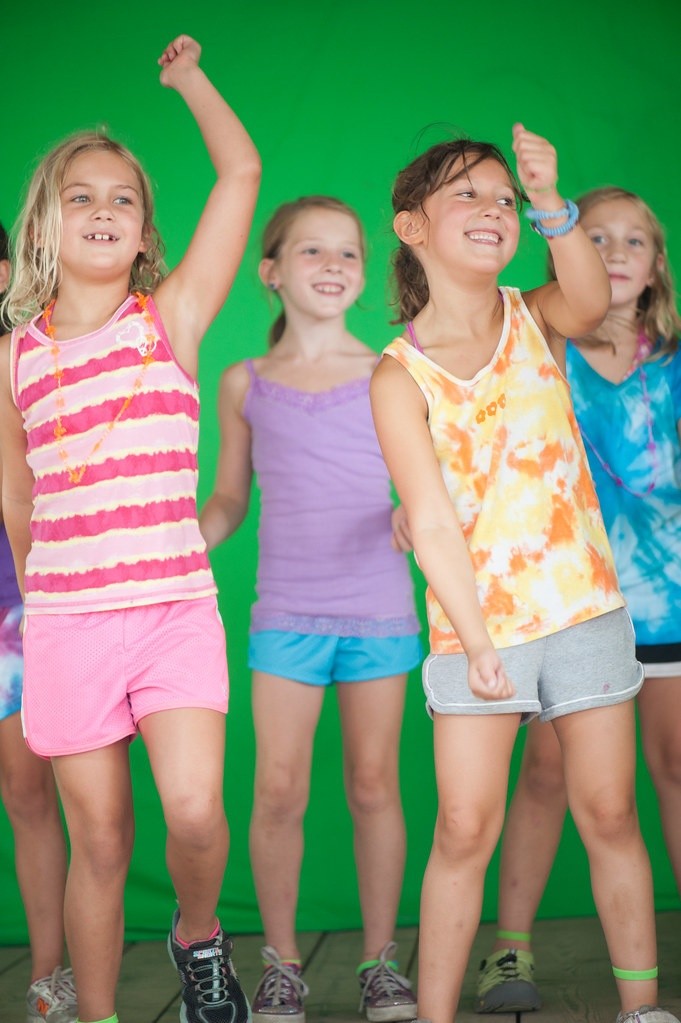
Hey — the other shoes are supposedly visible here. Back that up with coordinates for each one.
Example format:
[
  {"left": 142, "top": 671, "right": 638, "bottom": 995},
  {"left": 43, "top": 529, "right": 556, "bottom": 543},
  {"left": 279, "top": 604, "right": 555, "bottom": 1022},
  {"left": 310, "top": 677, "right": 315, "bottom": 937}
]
[{"left": 616, "top": 1006, "right": 679, "bottom": 1023}]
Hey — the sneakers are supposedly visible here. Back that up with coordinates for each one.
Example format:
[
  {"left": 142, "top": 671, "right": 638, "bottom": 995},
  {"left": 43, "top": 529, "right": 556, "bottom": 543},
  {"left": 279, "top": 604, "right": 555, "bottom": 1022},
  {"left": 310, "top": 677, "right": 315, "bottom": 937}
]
[
  {"left": 354, "top": 940, "right": 419, "bottom": 1021},
  {"left": 475, "top": 947, "right": 540, "bottom": 1015},
  {"left": 25, "top": 966, "right": 74, "bottom": 1023},
  {"left": 167, "top": 906, "right": 247, "bottom": 1023},
  {"left": 249, "top": 945, "right": 308, "bottom": 1023}
]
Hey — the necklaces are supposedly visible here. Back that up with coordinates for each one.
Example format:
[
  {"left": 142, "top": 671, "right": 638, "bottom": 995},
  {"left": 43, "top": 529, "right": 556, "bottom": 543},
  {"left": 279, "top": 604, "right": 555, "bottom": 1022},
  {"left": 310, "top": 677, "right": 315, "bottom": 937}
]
[
  {"left": 576, "top": 323, "right": 659, "bottom": 498},
  {"left": 45, "top": 290, "right": 155, "bottom": 484}
]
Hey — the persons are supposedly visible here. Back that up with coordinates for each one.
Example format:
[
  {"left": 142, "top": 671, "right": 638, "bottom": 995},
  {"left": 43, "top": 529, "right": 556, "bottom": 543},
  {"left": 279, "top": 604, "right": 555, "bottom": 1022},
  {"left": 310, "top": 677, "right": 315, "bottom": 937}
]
[
  {"left": 387, "top": 193, "right": 681, "bottom": 1014},
  {"left": 198, "top": 197, "right": 420, "bottom": 1023},
  {"left": 381, "top": 124, "right": 680, "bottom": 1022},
  {"left": 1, "top": 35, "right": 261, "bottom": 1023}
]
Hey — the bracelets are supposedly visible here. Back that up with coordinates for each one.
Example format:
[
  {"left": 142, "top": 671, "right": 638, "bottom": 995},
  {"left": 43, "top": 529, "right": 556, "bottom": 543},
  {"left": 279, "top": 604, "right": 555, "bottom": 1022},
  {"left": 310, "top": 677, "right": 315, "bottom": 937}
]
[{"left": 527, "top": 199, "right": 578, "bottom": 238}]
